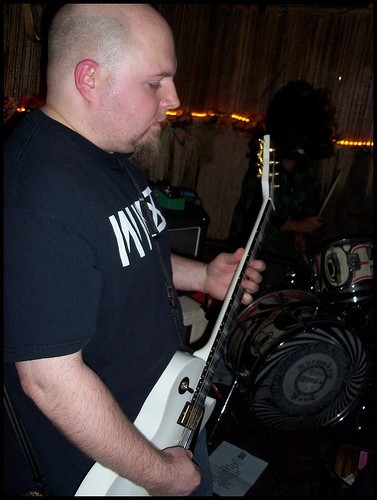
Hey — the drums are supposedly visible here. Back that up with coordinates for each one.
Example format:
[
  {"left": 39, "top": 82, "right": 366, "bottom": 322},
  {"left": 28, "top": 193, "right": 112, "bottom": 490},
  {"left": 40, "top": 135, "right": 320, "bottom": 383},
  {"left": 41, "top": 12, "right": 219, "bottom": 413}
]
[
  {"left": 221, "top": 287, "right": 370, "bottom": 433},
  {"left": 309, "top": 231, "right": 377, "bottom": 306}
]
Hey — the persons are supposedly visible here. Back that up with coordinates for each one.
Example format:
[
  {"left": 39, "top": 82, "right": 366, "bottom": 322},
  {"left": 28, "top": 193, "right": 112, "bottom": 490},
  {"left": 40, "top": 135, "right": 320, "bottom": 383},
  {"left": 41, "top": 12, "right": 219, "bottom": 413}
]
[
  {"left": 227, "top": 139, "right": 325, "bottom": 262},
  {"left": 0, "top": 4, "right": 265, "bottom": 498}
]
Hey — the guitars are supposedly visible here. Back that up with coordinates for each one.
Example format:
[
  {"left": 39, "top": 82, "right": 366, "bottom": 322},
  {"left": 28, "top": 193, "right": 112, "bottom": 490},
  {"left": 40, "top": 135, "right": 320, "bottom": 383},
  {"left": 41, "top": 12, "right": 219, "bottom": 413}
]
[{"left": 72, "top": 133, "right": 282, "bottom": 496}]
